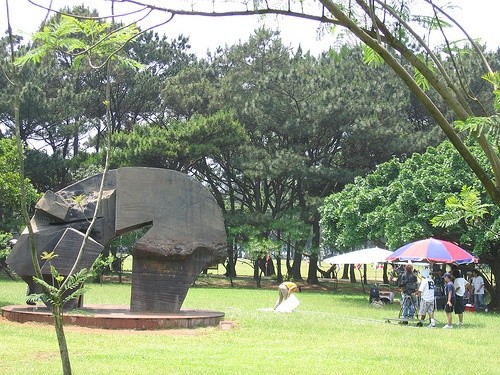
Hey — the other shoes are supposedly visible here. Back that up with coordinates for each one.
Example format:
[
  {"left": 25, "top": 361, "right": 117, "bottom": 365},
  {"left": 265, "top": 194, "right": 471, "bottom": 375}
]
[
  {"left": 428, "top": 323, "right": 436, "bottom": 328},
  {"left": 485, "top": 308, "right": 488, "bottom": 313},
  {"left": 442, "top": 324, "right": 454, "bottom": 329},
  {"left": 456, "top": 322, "right": 464, "bottom": 326},
  {"left": 416, "top": 321, "right": 424, "bottom": 326},
  {"left": 409, "top": 315, "right": 415, "bottom": 319},
  {"left": 402, "top": 320, "right": 410, "bottom": 324}
]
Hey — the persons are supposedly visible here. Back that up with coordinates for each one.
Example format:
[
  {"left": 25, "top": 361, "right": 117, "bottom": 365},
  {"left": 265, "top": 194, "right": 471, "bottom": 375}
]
[
  {"left": 397, "top": 264, "right": 488, "bottom": 329},
  {"left": 274, "top": 281, "right": 301, "bottom": 310}
]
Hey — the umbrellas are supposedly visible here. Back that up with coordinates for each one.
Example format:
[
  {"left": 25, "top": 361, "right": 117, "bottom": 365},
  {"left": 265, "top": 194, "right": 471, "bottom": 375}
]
[
  {"left": 323, "top": 246, "right": 394, "bottom": 285},
  {"left": 385, "top": 237, "right": 478, "bottom": 272}
]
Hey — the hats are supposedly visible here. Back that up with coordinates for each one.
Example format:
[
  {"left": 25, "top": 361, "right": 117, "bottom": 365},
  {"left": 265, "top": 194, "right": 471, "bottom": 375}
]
[
  {"left": 442, "top": 273, "right": 451, "bottom": 278},
  {"left": 421, "top": 270, "right": 430, "bottom": 277}
]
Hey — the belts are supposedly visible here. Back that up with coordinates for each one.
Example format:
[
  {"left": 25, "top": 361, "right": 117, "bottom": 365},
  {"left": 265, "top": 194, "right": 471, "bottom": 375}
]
[{"left": 281, "top": 283, "right": 289, "bottom": 290}]
